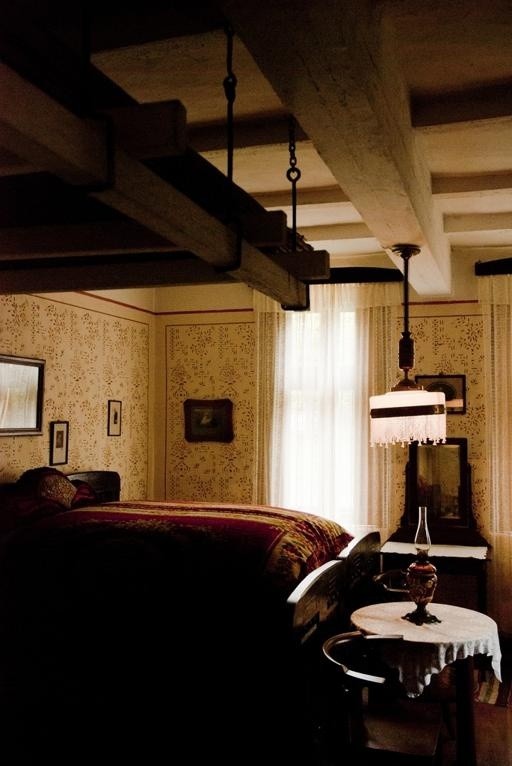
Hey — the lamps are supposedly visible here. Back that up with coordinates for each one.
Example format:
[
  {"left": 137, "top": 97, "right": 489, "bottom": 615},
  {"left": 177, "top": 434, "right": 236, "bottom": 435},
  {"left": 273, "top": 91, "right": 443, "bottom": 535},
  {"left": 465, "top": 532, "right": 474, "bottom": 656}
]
[
  {"left": 404, "top": 506, "right": 439, "bottom": 625},
  {"left": 370, "top": 248, "right": 447, "bottom": 449}
]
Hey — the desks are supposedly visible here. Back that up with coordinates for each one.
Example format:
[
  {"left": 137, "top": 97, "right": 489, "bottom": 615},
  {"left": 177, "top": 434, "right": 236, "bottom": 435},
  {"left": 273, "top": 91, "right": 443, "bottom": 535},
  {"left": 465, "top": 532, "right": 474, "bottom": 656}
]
[{"left": 351, "top": 601, "right": 503, "bottom": 715}]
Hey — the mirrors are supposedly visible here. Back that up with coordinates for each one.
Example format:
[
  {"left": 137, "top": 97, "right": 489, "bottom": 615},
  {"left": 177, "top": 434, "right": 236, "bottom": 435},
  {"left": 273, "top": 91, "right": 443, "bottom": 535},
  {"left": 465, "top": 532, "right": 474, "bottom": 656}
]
[{"left": 407, "top": 438, "right": 469, "bottom": 530}]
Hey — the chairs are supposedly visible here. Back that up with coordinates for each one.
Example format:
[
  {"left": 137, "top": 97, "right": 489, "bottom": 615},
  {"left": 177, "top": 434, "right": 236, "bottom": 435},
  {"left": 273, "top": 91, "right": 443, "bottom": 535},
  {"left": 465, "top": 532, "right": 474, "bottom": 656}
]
[{"left": 321, "top": 568, "right": 469, "bottom": 764}]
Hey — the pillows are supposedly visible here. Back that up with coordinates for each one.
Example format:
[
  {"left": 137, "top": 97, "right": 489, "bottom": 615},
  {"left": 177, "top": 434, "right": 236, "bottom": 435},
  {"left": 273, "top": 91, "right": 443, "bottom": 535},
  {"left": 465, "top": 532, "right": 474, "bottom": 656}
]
[{"left": 2, "top": 467, "right": 96, "bottom": 529}]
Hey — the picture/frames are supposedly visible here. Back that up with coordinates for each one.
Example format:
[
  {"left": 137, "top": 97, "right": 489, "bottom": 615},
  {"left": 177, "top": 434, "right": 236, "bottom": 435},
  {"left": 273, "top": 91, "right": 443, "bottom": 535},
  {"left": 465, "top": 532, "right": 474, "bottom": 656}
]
[
  {"left": 185, "top": 399, "right": 233, "bottom": 442},
  {"left": 50, "top": 421, "right": 68, "bottom": 464},
  {"left": 416, "top": 375, "right": 466, "bottom": 414},
  {"left": 0, "top": 354, "right": 46, "bottom": 436},
  {"left": 108, "top": 400, "right": 122, "bottom": 436}
]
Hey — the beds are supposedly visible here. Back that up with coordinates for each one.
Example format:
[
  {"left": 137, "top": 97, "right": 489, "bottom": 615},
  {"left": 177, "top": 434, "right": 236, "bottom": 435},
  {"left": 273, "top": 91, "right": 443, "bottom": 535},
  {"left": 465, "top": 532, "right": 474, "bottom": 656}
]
[{"left": 1, "top": 471, "right": 381, "bottom": 645}]
